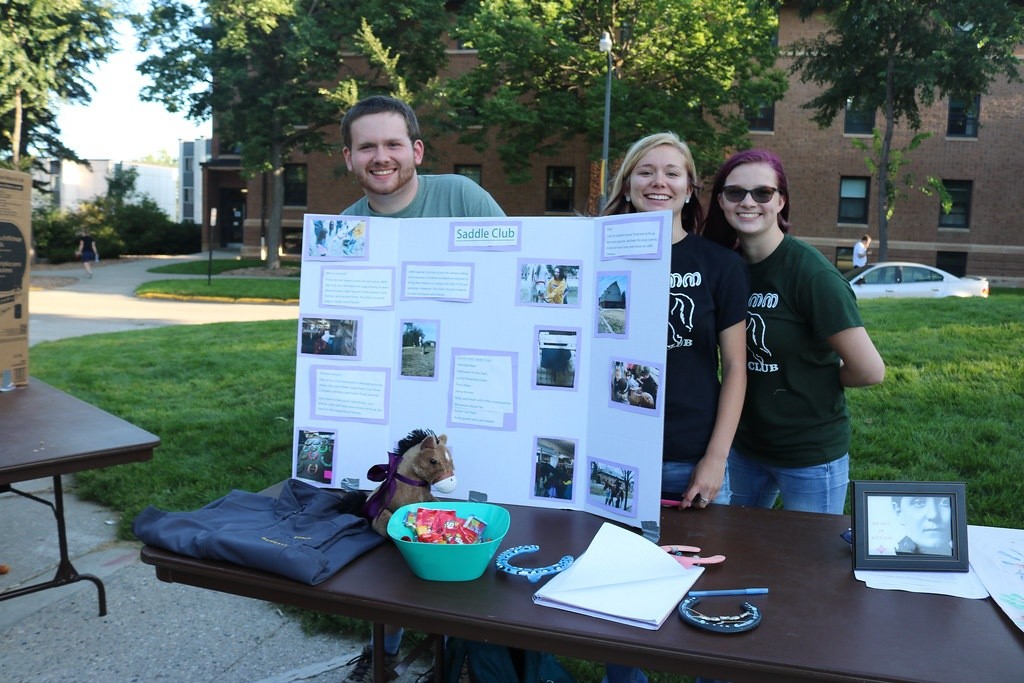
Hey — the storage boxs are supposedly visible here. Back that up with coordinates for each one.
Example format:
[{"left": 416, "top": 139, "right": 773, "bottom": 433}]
[{"left": 0, "top": 169, "right": 32, "bottom": 392}]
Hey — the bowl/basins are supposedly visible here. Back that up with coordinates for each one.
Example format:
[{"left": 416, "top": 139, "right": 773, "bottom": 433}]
[{"left": 386, "top": 502, "right": 511, "bottom": 582}]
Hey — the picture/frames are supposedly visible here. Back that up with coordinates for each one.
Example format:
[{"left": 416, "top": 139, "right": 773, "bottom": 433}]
[{"left": 851, "top": 479, "right": 968, "bottom": 572}]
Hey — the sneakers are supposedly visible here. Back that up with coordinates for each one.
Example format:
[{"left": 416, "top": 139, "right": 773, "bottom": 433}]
[
  {"left": 341, "top": 645, "right": 400, "bottom": 683},
  {"left": 415, "top": 656, "right": 469, "bottom": 683}
]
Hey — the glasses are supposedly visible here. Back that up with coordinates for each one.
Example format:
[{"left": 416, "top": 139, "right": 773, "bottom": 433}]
[{"left": 719, "top": 185, "right": 781, "bottom": 203}]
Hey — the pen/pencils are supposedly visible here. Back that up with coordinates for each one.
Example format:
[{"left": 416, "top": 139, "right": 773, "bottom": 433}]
[{"left": 689, "top": 588, "right": 769, "bottom": 597}]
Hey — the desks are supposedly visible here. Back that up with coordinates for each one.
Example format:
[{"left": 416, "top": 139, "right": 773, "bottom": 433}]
[
  {"left": 0, "top": 377, "right": 162, "bottom": 617},
  {"left": 140, "top": 478, "right": 1024, "bottom": 683}
]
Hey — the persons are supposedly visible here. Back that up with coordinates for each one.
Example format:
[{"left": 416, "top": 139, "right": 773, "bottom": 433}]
[
  {"left": 605, "top": 489, "right": 613, "bottom": 506},
  {"left": 611, "top": 363, "right": 657, "bottom": 408},
  {"left": 418, "top": 334, "right": 422, "bottom": 348},
  {"left": 75, "top": 229, "right": 98, "bottom": 278},
  {"left": 592, "top": 462, "right": 598, "bottom": 475},
  {"left": 851, "top": 235, "right": 872, "bottom": 284},
  {"left": 888, "top": 496, "right": 954, "bottom": 558},
  {"left": 591, "top": 132, "right": 747, "bottom": 508},
  {"left": 314, "top": 219, "right": 368, "bottom": 254},
  {"left": 701, "top": 141, "right": 888, "bottom": 521},
  {"left": 312, "top": 323, "right": 342, "bottom": 355},
  {"left": 545, "top": 266, "right": 568, "bottom": 304},
  {"left": 615, "top": 493, "right": 621, "bottom": 509},
  {"left": 330, "top": 96, "right": 506, "bottom": 218}
]
